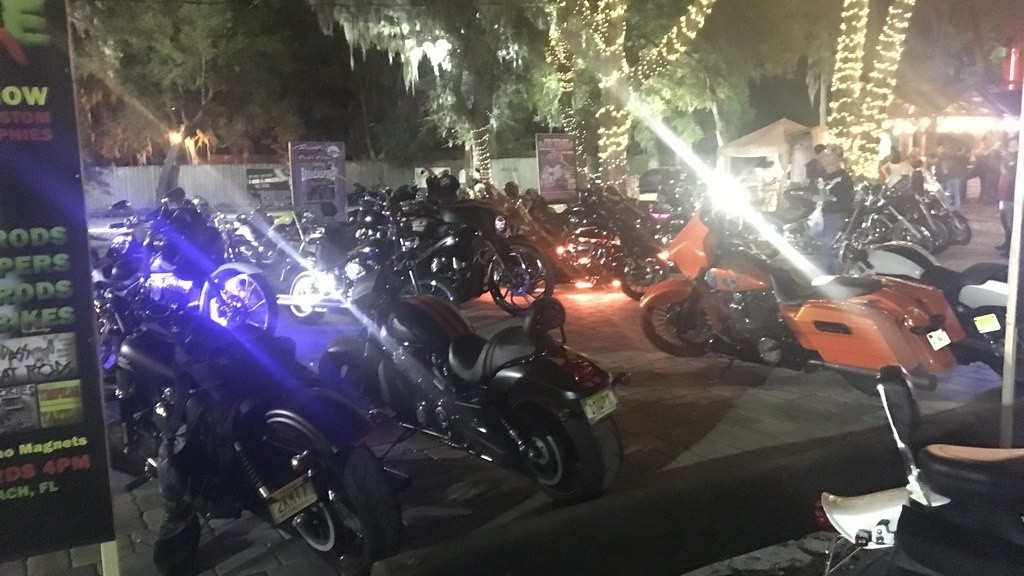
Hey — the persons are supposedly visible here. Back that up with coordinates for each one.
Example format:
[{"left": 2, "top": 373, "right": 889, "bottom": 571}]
[
  {"left": 805, "top": 144, "right": 828, "bottom": 189},
  {"left": 819, "top": 144, "right": 854, "bottom": 275},
  {"left": 877, "top": 128, "right": 1019, "bottom": 260},
  {"left": 153, "top": 186, "right": 224, "bottom": 287}
]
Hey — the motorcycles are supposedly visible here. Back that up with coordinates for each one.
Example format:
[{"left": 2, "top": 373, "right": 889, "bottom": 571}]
[
  {"left": 634, "top": 179, "right": 963, "bottom": 400},
  {"left": 91, "top": 150, "right": 968, "bottom": 316},
  {"left": 809, "top": 205, "right": 1022, "bottom": 395},
  {"left": 805, "top": 362, "right": 1023, "bottom": 574},
  {"left": 304, "top": 236, "right": 624, "bottom": 504},
  {"left": 88, "top": 253, "right": 415, "bottom": 574}
]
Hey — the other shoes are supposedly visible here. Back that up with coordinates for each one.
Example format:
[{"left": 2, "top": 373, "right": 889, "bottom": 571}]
[{"left": 995, "top": 240, "right": 1011, "bottom": 261}]
